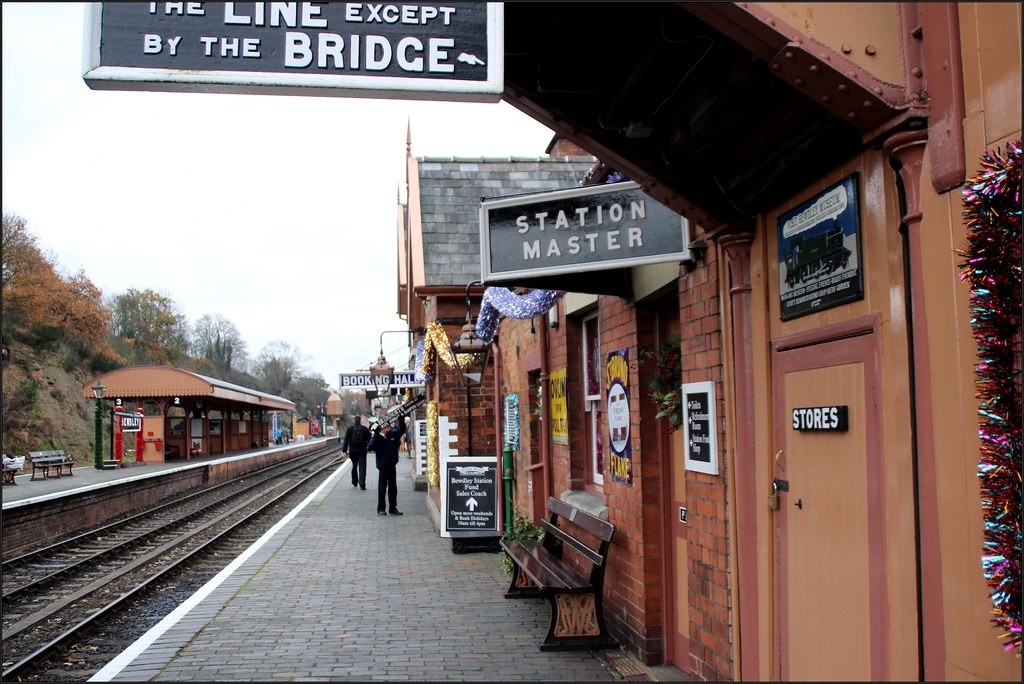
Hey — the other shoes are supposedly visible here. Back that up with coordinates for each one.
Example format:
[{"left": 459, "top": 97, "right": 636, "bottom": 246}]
[
  {"left": 354, "top": 484, "right": 357, "bottom": 487},
  {"left": 389, "top": 508, "right": 403, "bottom": 515},
  {"left": 361, "top": 487, "right": 366, "bottom": 490},
  {"left": 379, "top": 511, "right": 387, "bottom": 515}
]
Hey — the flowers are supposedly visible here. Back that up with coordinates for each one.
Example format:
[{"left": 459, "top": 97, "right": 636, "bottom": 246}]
[{"left": 639, "top": 334, "right": 684, "bottom": 434}]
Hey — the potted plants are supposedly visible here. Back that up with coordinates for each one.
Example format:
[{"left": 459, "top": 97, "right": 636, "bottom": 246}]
[{"left": 499, "top": 497, "right": 563, "bottom": 587}]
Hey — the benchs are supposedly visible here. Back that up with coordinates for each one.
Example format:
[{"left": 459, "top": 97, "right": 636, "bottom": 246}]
[
  {"left": 165, "top": 443, "right": 180, "bottom": 461},
  {"left": 499, "top": 495, "right": 622, "bottom": 651},
  {"left": 28, "top": 449, "right": 75, "bottom": 480}
]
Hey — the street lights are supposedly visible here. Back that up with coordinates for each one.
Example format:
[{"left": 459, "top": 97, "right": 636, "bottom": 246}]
[{"left": 90, "top": 378, "right": 107, "bottom": 470}]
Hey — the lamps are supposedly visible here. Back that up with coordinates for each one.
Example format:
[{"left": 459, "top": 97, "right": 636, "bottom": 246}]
[
  {"left": 370, "top": 327, "right": 427, "bottom": 398},
  {"left": 448, "top": 279, "right": 535, "bottom": 388}
]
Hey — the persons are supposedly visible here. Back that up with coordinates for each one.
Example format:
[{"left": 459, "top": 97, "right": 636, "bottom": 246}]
[
  {"left": 368, "top": 416, "right": 407, "bottom": 515},
  {"left": 342, "top": 416, "right": 371, "bottom": 490},
  {"left": 276, "top": 426, "right": 289, "bottom": 445}
]
